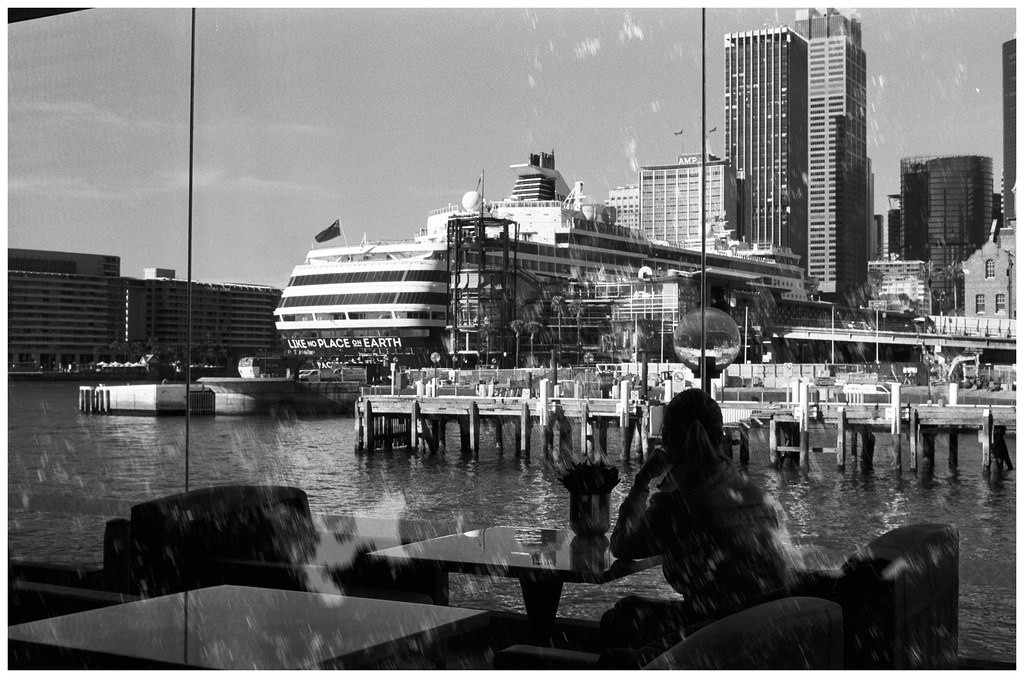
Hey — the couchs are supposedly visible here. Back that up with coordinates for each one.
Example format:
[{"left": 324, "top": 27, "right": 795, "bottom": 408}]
[
  {"left": 492, "top": 595, "right": 841, "bottom": 670},
  {"left": 104, "top": 485, "right": 450, "bottom": 609},
  {"left": 749, "top": 521, "right": 959, "bottom": 670}
]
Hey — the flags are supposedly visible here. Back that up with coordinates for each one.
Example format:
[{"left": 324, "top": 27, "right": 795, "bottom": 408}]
[{"left": 314, "top": 220, "right": 340, "bottom": 243}]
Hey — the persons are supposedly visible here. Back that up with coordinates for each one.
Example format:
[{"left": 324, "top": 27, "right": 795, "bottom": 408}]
[{"left": 598, "top": 387, "right": 799, "bottom": 670}]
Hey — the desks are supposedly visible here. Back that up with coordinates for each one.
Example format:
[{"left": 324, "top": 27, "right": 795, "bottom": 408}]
[
  {"left": 364, "top": 527, "right": 660, "bottom": 643},
  {"left": 8, "top": 580, "right": 488, "bottom": 670}
]
